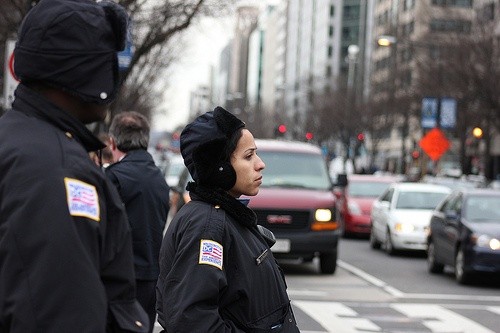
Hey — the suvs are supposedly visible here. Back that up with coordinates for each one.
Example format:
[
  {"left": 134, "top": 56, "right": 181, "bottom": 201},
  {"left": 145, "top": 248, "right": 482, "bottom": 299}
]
[{"left": 177, "top": 136, "right": 349, "bottom": 275}]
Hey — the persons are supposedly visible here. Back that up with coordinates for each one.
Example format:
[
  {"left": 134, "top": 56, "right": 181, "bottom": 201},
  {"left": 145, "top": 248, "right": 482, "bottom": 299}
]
[
  {"left": 105, "top": 112, "right": 169, "bottom": 333},
  {"left": 155, "top": 105, "right": 300, "bottom": 333},
  {"left": 0, "top": 0, "right": 149, "bottom": 333}
]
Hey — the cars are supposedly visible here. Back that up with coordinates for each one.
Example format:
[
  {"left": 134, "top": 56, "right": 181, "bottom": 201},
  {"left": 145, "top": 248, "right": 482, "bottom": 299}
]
[
  {"left": 426, "top": 186, "right": 500, "bottom": 285},
  {"left": 332, "top": 173, "right": 406, "bottom": 241},
  {"left": 369, "top": 181, "right": 453, "bottom": 257},
  {"left": 147, "top": 150, "right": 500, "bottom": 218}
]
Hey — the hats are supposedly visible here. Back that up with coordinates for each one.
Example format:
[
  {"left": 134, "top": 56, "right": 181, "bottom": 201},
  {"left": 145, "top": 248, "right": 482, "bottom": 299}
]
[
  {"left": 179, "top": 107, "right": 246, "bottom": 191},
  {"left": 15, "top": 0, "right": 128, "bottom": 104}
]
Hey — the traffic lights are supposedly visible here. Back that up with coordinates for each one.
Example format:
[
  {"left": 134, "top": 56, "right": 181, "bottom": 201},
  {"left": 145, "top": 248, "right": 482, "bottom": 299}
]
[
  {"left": 357, "top": 128, "right": 365, "bottom": 145},
  {"left": 274, "top": 121, "right": 286, "bottom": 137},
  {"left": 304, "top": 129, "right": 313, "bottom": 144}
]
